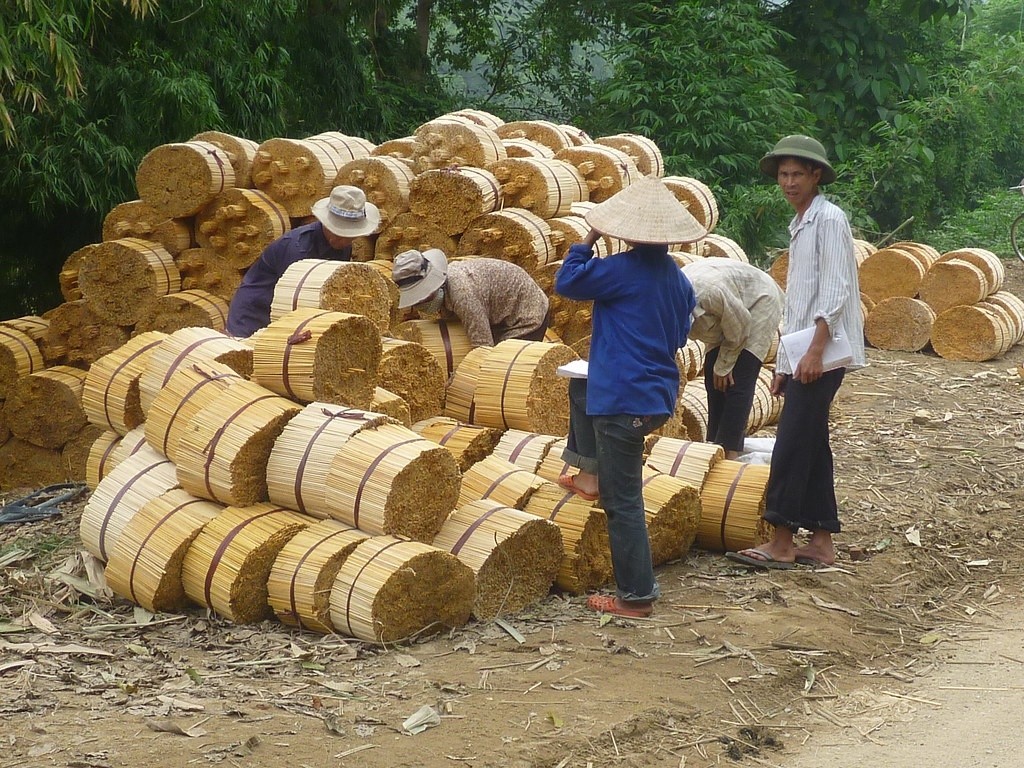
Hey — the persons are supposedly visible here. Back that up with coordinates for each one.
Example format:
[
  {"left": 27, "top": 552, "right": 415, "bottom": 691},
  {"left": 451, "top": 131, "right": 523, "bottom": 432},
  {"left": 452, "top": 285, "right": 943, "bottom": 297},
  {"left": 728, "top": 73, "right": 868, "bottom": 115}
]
[
  {"left": 724, "top": 133, "right": 865, "bottom": 570},
  {"left": 390, "top": 249, "right": 550, "bottom": 347},
  {"left": 680, "top": 256, "right": 786, "bottom": 460},
  {"left": 225, "top": 183, "right": 380, "bottom": 341},
  {"left": 559, "top": 175, "right": 708, "bottom": 620}
]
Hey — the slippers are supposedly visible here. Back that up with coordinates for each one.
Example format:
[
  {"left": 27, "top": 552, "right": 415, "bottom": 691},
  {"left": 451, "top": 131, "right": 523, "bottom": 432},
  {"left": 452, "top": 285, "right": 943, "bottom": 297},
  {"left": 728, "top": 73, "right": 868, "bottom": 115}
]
[
  {"left": 726, "top": 549, "right": 794, "bottom": 569},
  {"left": 558, "top": 475, "right": 599, "bottom": 500},
  {"left": 795, "top": 558, "right": 817, "bottom": 566},
  {"left": 587, "top": 595, "right": 648, "bottom": 617}
]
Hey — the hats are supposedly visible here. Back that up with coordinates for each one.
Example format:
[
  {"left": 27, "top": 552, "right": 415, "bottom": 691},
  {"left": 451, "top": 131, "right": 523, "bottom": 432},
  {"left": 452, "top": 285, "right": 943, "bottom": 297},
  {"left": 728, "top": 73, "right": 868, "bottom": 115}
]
[
  {"left": 585, "top": 173, "right": 708, "bottom": 245},
  {"left": 392, "top": 248, "right": 448, "bottom": 310},
  {"left": 759, "top": 134, "right": 837, "bottom": 185},
  {"left": 311, "top": 185, "right": 381, "bottom": 238}
]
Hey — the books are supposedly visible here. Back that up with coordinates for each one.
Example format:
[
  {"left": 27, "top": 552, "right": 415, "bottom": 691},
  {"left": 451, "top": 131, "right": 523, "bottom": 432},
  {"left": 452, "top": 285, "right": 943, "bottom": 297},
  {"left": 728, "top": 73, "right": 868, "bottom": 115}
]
[{"left": 779, "top": 319, "right": 853, "bottom": 379}]
